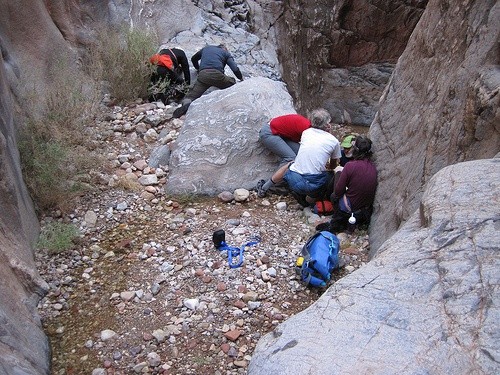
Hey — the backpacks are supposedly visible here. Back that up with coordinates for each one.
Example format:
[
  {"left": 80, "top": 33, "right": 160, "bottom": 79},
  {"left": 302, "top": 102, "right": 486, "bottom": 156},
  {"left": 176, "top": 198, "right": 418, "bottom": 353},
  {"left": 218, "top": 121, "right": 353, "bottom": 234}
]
[{"left": 302, "top": 231, "right": 339, "bottom": 286}]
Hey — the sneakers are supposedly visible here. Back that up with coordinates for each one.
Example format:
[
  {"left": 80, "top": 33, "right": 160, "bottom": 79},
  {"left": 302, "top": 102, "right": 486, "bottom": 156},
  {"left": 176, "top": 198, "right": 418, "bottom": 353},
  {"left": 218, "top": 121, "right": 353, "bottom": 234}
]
[
  {"left": 268, "top": 184, "right": 288, "bottom": 196},
  {"left": 257, "top": 179, "right": 266, "bottom": 198}
]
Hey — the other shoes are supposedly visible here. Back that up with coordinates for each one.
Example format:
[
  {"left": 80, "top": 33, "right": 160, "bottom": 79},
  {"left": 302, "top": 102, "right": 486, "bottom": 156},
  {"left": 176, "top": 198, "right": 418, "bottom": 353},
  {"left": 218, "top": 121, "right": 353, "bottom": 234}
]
[
  {"left": 173, "top": 103, "right": 190, "bottom": 118},
  {"left": 292, "top": 191, "right": 309, "bottom": 207}
]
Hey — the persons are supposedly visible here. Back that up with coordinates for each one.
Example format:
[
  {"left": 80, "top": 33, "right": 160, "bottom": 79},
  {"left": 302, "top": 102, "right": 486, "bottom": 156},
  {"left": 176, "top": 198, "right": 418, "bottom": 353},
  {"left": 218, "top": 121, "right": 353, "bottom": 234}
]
[
  {"left": 256, "top": 114, "right": 311, "bottom": 198},
  {"left": 282, "top": 108, "right": 341, "bottom": 212},
  {"left": 146, "top": 46, "right": 190, "bottom": 105},
  {"left": 326, "top": 134, "right": 378, "bottom": 233},
  {"left": 173, "top": 43, "right": 244, "bottom": 118}
]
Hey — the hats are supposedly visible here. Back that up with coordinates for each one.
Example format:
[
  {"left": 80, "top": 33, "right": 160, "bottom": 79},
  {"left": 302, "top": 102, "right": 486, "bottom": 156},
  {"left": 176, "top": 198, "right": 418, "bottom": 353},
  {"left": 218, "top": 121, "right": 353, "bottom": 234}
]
[{"left": 341, "top": 135, "right": 355, "bottom": 148}]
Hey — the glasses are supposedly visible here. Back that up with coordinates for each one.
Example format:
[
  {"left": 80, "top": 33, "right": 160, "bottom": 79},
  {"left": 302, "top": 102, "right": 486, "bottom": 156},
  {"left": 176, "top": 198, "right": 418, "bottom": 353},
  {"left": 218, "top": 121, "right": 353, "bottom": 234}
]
[{"left": 344, "top": 145, "right": 353, "bottom": 150}]
